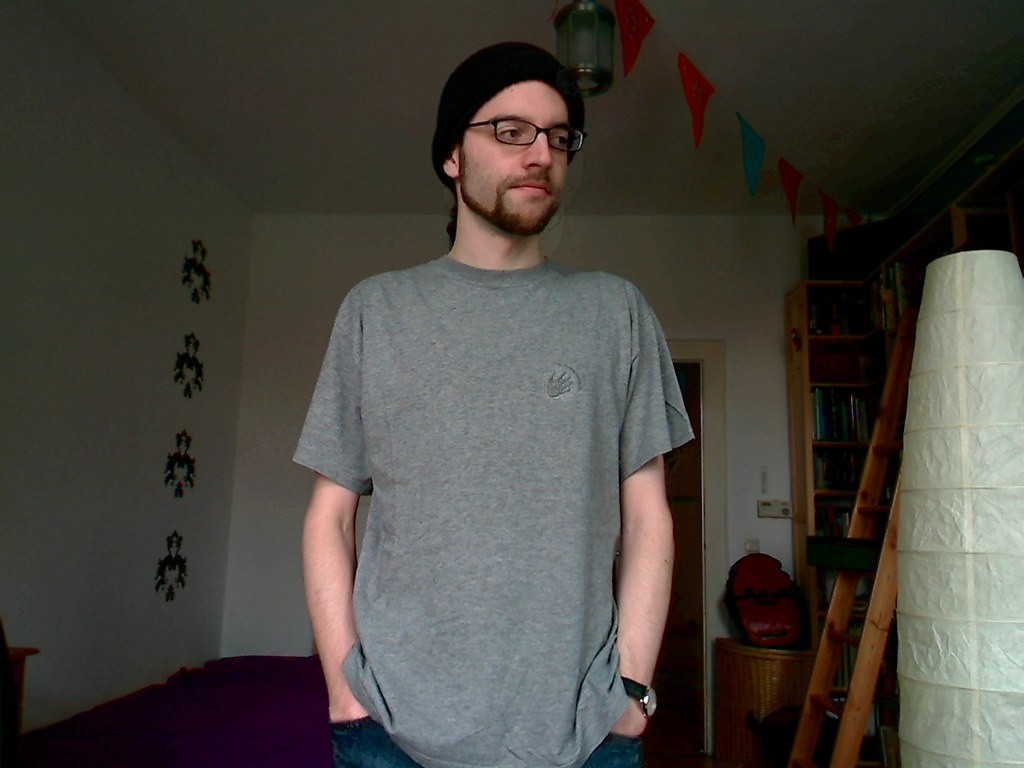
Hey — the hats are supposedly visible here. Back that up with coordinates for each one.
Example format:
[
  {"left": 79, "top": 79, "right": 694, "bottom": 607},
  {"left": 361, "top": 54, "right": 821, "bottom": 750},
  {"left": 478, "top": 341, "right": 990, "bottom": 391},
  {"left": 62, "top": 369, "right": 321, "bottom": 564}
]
[{"left": 431, "top": 41, "right": 586, "bottom": 197}]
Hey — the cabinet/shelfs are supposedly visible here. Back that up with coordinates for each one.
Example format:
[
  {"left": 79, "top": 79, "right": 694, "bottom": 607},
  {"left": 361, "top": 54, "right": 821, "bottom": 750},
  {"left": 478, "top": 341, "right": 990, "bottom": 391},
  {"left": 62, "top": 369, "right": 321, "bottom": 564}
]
[{"left": 784, "top": 281, "right": 886, "bottom": 692}]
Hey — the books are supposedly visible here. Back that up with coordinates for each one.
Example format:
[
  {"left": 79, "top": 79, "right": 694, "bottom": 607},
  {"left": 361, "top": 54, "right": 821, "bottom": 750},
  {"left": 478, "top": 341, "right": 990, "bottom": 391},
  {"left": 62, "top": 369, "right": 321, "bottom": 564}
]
[
  {"left": 812, "top": 389, "right": 869, "bottom": 442},
  {"left": 814, "top": 453, "right": 863, "bottom": 491},
  {"left": 879, "top": 262, "right": 909, "bottom": 331}
]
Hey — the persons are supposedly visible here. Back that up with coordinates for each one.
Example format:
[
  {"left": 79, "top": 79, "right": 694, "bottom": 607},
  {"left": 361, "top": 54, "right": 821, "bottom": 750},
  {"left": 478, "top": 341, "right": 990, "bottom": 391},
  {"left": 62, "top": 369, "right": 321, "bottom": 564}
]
[{"left": 290, "top": 42, "right": 694, "bottom": 768}]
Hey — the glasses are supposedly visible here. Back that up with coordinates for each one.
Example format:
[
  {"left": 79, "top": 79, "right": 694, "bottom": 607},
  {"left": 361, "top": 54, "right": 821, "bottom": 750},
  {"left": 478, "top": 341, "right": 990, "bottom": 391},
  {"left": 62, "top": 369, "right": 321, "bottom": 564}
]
[{"left": 467, "top": 119, "right": 589, "bottom": 152}]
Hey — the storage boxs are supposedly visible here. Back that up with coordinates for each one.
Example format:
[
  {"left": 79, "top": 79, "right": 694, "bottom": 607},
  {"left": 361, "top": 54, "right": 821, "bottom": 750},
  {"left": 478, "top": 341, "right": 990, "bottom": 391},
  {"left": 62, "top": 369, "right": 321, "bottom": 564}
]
[{"left": 714, "top": 637, "right": 817, "bottom": 768}]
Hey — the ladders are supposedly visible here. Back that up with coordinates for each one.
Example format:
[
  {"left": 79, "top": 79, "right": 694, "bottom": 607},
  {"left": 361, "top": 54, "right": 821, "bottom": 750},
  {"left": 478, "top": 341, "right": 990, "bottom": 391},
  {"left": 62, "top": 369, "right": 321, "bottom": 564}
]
[{"left": 788, "top": 306, "right": 915, "bottom": 768}]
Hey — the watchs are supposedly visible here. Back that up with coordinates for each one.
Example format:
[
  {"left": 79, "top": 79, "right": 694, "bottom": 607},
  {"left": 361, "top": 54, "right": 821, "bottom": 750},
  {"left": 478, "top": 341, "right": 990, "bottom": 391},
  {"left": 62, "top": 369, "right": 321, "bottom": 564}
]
[{"left": 621, "top": 676, "right": 657, "bottom": 719}]
